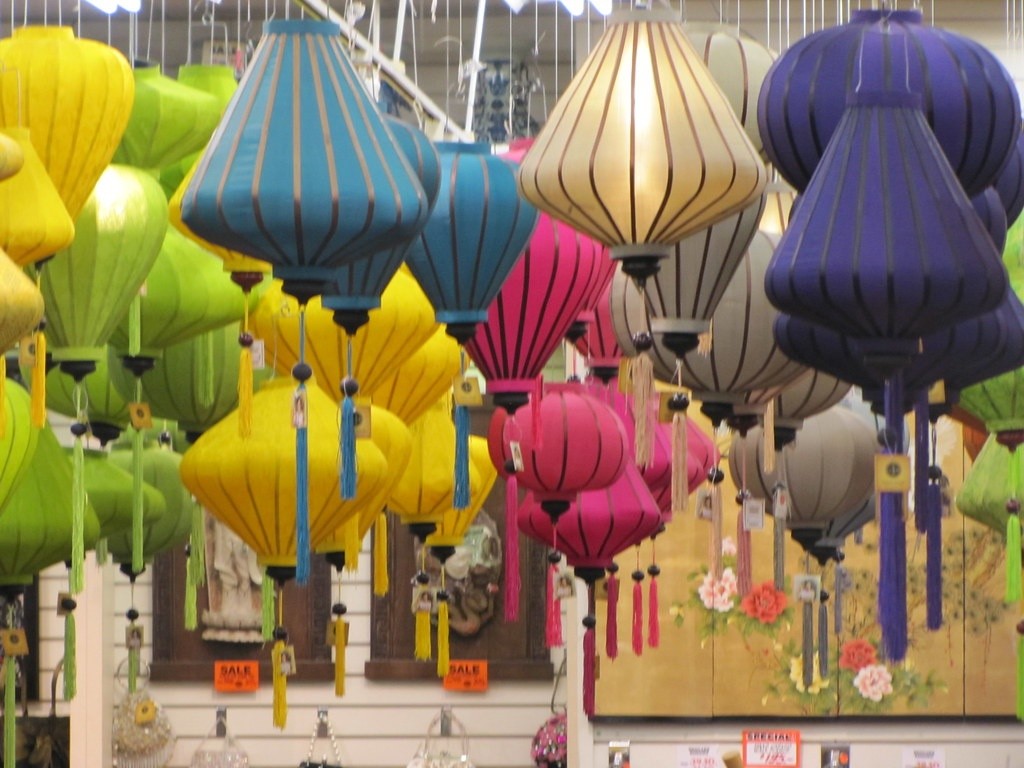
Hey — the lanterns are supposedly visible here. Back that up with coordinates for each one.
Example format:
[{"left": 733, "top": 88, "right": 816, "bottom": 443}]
[{"left": 0, "top": 10, "right": 1024, "bottom": 768}]
